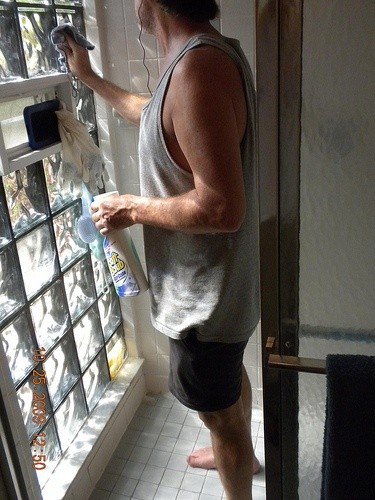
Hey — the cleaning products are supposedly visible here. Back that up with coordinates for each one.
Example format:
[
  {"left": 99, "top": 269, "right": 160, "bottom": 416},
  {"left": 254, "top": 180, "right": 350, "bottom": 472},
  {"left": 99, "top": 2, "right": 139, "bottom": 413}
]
[{"left": 89, "top": 191, "right": 149, "bottom": 297}]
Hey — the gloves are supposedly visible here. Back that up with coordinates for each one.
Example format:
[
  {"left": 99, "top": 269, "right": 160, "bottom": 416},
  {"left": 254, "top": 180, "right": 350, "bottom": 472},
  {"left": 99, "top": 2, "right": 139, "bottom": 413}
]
[{"left": 56, "top": 109, "right": 110, "bottom": 198}]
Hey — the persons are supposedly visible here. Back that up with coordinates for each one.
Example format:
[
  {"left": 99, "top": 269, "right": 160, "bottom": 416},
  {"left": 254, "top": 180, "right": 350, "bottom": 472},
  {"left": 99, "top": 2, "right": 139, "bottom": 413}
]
[{"left": 65, "top": 0, "right": 263, "bottom": 500}]
